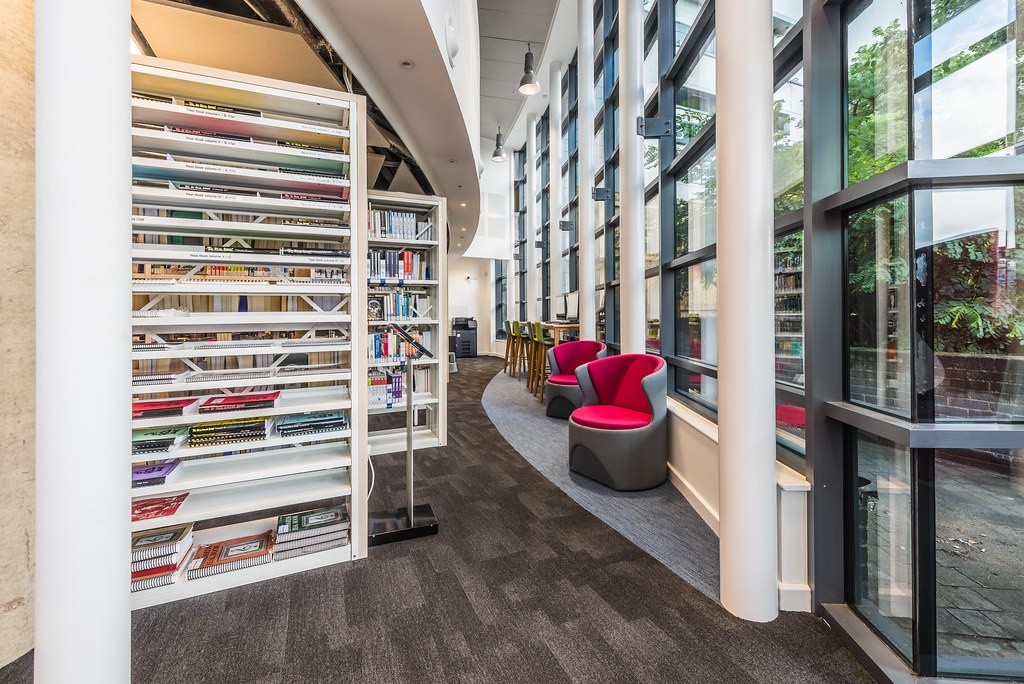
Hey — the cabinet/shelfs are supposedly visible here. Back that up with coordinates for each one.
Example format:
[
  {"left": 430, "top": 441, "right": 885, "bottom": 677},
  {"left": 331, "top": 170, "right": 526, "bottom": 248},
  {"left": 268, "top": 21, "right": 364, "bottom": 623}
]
[
  {"left": 368, "top": 191, "right": 449, "bottom": 456},
  {"left": 131, "top": 53, "right": 367, "bottom": 610}
]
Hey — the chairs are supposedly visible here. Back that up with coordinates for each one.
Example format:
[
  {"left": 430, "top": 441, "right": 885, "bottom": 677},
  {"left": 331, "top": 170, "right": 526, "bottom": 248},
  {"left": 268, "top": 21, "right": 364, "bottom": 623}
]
[
  {"left": 569, "top": 354, "right": 667, "bottom": 491},
  {"left": 545, "top": 340, "right": 607, "bottom": 418}
]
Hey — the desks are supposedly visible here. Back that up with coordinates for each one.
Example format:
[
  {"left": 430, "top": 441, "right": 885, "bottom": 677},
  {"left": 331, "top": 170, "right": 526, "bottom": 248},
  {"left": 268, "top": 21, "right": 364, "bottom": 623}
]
[{"left": 519, "top": 322, "right": 579, "bottom": 345}]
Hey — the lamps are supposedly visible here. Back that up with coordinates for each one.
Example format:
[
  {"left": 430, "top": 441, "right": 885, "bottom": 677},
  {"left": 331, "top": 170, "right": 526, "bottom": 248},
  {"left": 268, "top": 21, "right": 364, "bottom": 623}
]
[
  {"left": 518, "top": 42, "right": 540, "bottom": 95},
  {"left": 491, "top": 126, "right": 507, "bottom": 162}
]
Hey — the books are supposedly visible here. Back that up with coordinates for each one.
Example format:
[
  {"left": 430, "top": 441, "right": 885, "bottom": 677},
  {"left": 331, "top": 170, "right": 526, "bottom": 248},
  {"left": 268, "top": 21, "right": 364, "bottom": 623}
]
[
  {"left": 645, "top": 194, "right": 806, "bottom": 400},
  {"left": 132, "top": 84, "right": 435, "bottom": 595}
]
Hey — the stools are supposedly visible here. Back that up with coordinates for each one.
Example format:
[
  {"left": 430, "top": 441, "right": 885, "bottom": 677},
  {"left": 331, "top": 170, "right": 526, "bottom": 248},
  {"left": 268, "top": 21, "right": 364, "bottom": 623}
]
[
  {"left": 513, "top": 321, "right": 531, "bottom": 380},
  {"left": 504, "top": 321, "right": 525, "bottom": 376},
  {"left": 527, "top": 322, "right": 554, "bottom": 392},
  {"left": 533, "top": 322, "right": 568, "bottom": 402}
]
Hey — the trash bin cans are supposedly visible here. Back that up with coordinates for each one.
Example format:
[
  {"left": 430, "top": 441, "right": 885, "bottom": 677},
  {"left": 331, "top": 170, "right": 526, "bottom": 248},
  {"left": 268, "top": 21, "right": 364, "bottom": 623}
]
[{"left": 451, "top": 316, "right": 478, "bottom": 357}]
